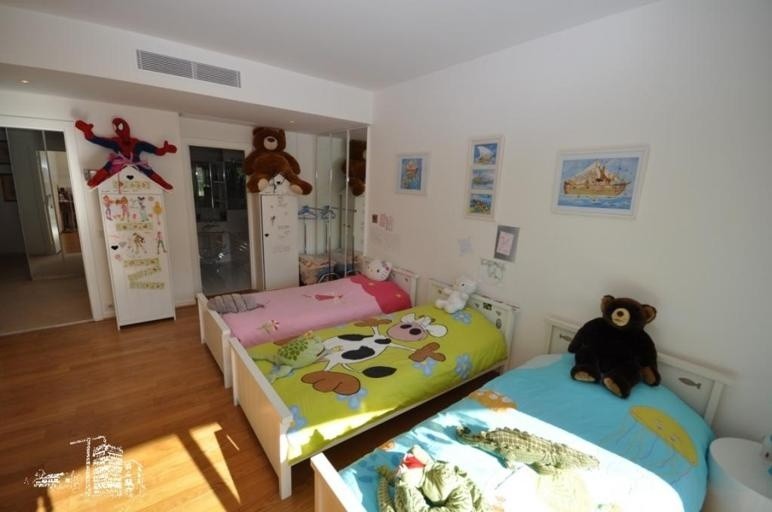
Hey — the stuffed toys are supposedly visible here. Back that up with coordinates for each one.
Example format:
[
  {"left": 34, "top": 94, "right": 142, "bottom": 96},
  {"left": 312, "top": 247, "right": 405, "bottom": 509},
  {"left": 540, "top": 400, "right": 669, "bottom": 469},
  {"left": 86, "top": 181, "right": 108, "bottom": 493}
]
[
  {"left": 435, "top": 279, "right": 477, "bottom": 312},
  {"left": 455, "top": 425, "right": 601, "bottom": 481},
  {"left": 373, "top": 445, "right": 484, "bottom": 512},
  {"left": 340, "top": 139, "right": 366, "bottom": 196},
  {"left": 251, "top": 330, "right": 342, "bottom": 384},
  {"left": 240, "top": 126, "right": 313, "bottom": 196},
  {"left": 364, "top": 258, "right": 392, "bottom": 282},
  {"left": 206, "top": 292, "right": 257, "bottom": 314},
  {"left": 569, "top": 294, "right": 665, "bottom": 401}
]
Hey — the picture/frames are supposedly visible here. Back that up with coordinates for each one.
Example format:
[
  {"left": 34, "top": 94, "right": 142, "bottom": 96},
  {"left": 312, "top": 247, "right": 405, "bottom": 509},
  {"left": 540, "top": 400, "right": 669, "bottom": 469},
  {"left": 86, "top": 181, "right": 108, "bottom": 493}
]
[
  {"left": 462, "top": 136, "right": 505, "bottom": 223},
  {"left": 0, "top": 173, "right": 17, "bottom": 202},
  {"left": 393, "top": 152, "right": 430, "bottom": 196},
  {"left": 549, "top": 144, "right": 650, "bottom": 220},
  {"left": 0, "top": 139, "right": 11, "bottom": 166},
  {"left": 493, "top": 224, "right": 520, "bottom": 263}
]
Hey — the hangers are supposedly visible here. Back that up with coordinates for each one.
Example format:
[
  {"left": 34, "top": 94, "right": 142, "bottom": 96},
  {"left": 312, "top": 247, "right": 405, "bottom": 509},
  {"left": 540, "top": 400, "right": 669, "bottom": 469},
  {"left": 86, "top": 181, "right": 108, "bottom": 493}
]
[
  {"left": 322, "top": 205, "right": 336, "bottom": 219},
  {"left": 298, "top": 205, "right": 318, "bottom": 220}
]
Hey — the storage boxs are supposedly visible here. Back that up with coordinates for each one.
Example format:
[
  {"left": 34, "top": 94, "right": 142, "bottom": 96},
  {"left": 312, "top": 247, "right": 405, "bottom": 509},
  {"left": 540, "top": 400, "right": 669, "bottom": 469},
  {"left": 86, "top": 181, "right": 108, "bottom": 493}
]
[{"left": 300, "top": 258, "right": 337, "bottom": 285}]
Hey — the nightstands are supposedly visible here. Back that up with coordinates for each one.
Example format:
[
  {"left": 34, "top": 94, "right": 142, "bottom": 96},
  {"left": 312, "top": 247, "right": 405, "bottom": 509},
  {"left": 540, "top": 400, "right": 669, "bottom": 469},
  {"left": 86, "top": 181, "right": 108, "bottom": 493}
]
[{"left": 702, "top": 436, "right": 772, "bottom": 512}]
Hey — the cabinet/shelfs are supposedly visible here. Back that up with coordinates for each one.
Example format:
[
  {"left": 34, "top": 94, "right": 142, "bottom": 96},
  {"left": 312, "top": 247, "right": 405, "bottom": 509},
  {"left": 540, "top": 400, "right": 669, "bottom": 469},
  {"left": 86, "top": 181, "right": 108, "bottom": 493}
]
[
  {"left": 254, "top": 170, "right": 300, "bottom": 291},
  {"left": 88, "top": 169, "right": 177, "bottom": 331}
]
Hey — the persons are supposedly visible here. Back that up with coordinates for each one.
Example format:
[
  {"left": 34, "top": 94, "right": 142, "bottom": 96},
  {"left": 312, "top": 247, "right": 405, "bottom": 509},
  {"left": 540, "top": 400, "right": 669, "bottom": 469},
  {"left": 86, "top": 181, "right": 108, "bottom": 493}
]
[{"left": 74, "top": 116, "right": 177, "bottom": 190}]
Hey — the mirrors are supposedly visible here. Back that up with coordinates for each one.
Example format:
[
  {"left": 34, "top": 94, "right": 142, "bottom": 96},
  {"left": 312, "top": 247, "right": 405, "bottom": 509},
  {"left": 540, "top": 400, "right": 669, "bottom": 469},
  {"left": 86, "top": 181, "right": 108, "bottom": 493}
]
[{"left": 314, "top": 125, "right": 370, "bottom": 280}]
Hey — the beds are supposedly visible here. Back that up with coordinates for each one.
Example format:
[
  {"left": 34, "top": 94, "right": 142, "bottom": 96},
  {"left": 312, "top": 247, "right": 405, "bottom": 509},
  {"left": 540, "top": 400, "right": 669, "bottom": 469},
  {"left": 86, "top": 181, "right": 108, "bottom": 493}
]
[
  {"left": 309, "top": 316, "right": 730, "bottom": 512},
  {"left": 194, "top": 256, "right": 420, "bottom": 390},
  {"left": 227, "top": 277, "right": 522, "bottom": 501}
]
